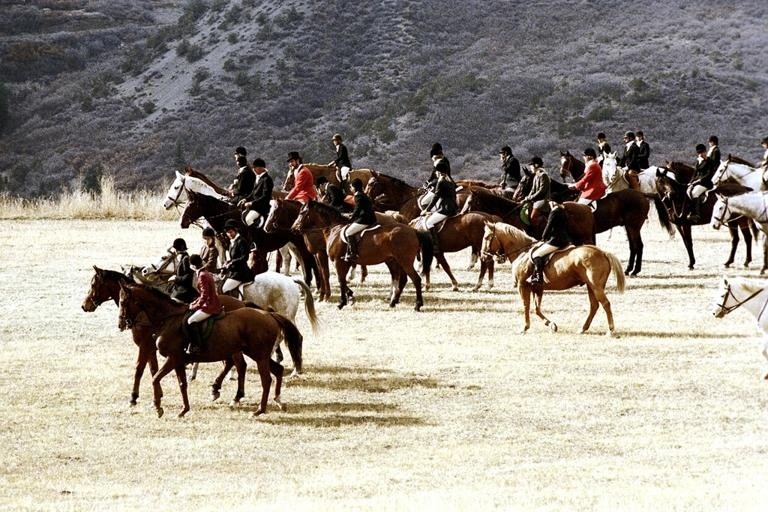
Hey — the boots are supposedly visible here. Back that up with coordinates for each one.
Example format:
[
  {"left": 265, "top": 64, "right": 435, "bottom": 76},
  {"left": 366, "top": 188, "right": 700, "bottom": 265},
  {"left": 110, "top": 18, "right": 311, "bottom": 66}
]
[
  {"left": 249, "top": 223, "right": 262, "bottom": 249},
  {"left": 686, "top": 198, "right": 700, "bottom": 220},
  {"left": 184, "top": 323, "right": 201, "bottom": 355},
  {"left": 532, "top": 258, "right": 543, "bottom": 283},
  {"left": 341, "top": 235, "right": 359, "bottom": 259},
  {"left": 430, "top": 227, "right": 440, "bottom": 253}
]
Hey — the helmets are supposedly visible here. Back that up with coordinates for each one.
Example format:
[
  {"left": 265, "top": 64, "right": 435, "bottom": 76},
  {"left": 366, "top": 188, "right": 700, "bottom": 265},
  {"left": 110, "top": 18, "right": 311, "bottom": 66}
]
[
  {"left": 529, "top": 156, "right": 543, "bottom": 164},
  {"left": 330, "top": 134, "right": 342, "bottom": 141},
  {"left": 695, "top": 136, "right": 717, "bottom": 153},
  {"left": 315, "top": 177, "right": 327, "bottom": 188},
  {"left": 353, "top": 179, "right": 362, "bottom": 190},
  {"left": 624, "top": 130, "right": 644, "bottom": 139},
  {"left": 429, "top": 142, "right": 449, "bottom": 173},
  {"left": 173, "top": 219, "right": 239, "bottom": 266},
  {"left": 760, "top": 137, "right": 767, "bottom": 144},
  {"left": 498, "top": 147, "right": 513, "bottom": 156},
  {"left": 581, "top": 148, "right": 593, "bottom": 156},
  {"left": 547, "top": 194, "right": 564, "bottom": 205},
  {"left": 596, "top": 134, "right": 606, "bottom": 139}
]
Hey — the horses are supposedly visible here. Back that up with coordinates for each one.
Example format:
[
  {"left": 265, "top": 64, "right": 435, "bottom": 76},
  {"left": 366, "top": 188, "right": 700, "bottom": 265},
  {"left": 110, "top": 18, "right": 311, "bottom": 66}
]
[
  {"left": 709, "top": 191, "right": 768, "bottom": 275},
  {"left": 289, "top": 198, "right": 423, "bottom": 311},
  {"left": 119, "top": 263, "right": 146, "bottom": 279},
  {"left": 477, "top": 219, "right": 627, "bottom": 339},
  {"left": 711, "top": 158, "right": 768, "bottom": 191},
  {"left": 559, "top": 150, "right": 586, "bottom": 182},
  {"left": 545, "top": 176, "right": 650, "bottom": 277},
  {"left": 161, "top": 163, "right": 321, "bottom": 293},
  {"left": 512, "top": 166, "right": 579, "bottom": 202},
  {"left": 283, "top": 161, "right": 378, "bottom": 191},
  {"left": 364, "top": 167, "right": 498, "bottom": 224},
  {"left": 391, "top": 187, "right": 501, "bottom": 292},
  {"left": 596, "top": 151, "right": 676, "bottom": 240},
  {"left": 117, "top": 282, "right": 306, "bottom": 418},
  {"left": 167, "top": 264, "right": 320, "bottom": 364},
  {"left": 460, "top": 186, "right": 597, "bottom": 246},
  {"left": 655, "top": 157, "right": 760, "bottom": 270},
  {"left": 183, "top": 162, "right": 289, "bottom": 200},
  {"left": 711, "top": 276, "right": 768, "bottom": 380},
  {"left": 80, "top": 264, "right": 276, "bottom": 407}
]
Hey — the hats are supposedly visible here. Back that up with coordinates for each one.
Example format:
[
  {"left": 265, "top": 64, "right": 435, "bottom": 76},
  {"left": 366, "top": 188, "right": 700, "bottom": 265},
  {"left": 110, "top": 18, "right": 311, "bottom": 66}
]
[
  {"left": 234, "top": 146, "right": 248, "bottom": 166},
  {"left": 252, "top": 159, "right": 266, "bottom": 168},
  {"left": 287, "top": 151, "right": 299, "bottom": 162}
]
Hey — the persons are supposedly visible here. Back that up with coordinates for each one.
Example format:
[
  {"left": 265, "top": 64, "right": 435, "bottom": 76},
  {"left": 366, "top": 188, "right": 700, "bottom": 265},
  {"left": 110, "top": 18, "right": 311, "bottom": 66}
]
[
  {"left": 314, "top": 176, "right": 344, "bottom": 210},
  {"left": 183, "top": 254, "right": 222, "bottom": 355},
  {"left": 524, "top": 193, "right": 570, "bottom": 284},
  {"left": 421, "top": 157, "right": 458, "bottom": 254},
  {"left": 327, "top": 134, "right": 351, "bottom": 195},
  {"left": 342, "top": 178, "right": 375, "bottom": 262},
  {"left": 757, "top": 136, "right": 768, "bottom": 195},
  {"left": 567, "top": 148, "right": 606, "bottom": 206},
  {"left": 519, "top": 156, "right": 551, "bottom": 218},
  {"left": 498, "top": 146, "right": 522, "bottom": 193},
  {"left": 421, "top": 143, "right": 450, "bottom": 211},
  {"left": 687, "top": 135, "right": 721, "bottom": 219},
  {"left": 166, "top": 238, "right": 200, "bottom": 307},
  {"left": 618, "top": 131, "right": 650, "bottom": 191},
  {"left": 222, "top": 218, "right": 250, "bottom": 295},
  {"left": 597, "top": 134, "right": 611, "bottom": 167},
  {"left": 283, "top": 152, "right": 316, "bottom": 204},
  {"left": 198, "top": 226, "right": 219, "bottom": 273},
  {"left": 227, "top": 147, "right": 274, "bottom": 253}
]
